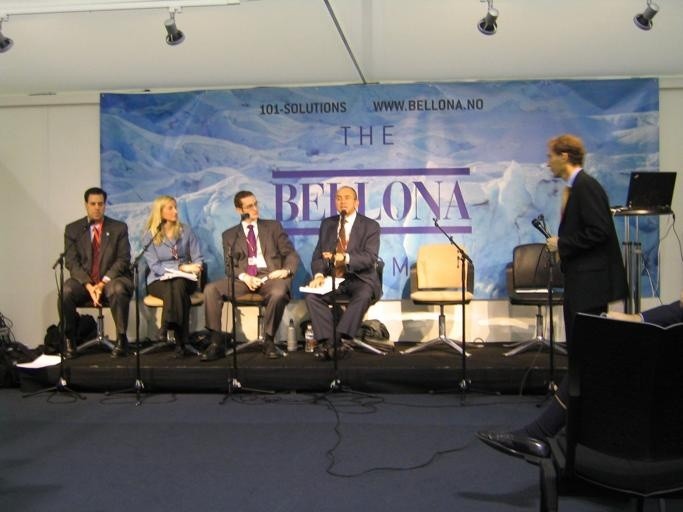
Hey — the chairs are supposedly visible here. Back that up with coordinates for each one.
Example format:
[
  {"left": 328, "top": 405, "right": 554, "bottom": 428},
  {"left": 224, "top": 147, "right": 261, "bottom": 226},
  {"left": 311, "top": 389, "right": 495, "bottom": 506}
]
[
  {"left": 322, "top": 259, "right": 387, "bottom": 355},
  {"left": 502, "top": 240, "right": 568, "bottom": 360},
  {"left": 399, "top": 243, "right": 483, "bottom": 358},
  {"left": 535, "top": 306, "right": 680, "bottom": 511}
]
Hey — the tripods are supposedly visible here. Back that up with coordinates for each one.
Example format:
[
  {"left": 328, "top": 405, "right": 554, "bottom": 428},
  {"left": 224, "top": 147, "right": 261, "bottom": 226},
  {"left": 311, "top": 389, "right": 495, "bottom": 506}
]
[
  {"left": 311, "top": 225, "right": 383, "bottom": 404},
  {"left": 535, "top": 215, "right": 561, "bottom": 408},
  {"left": 218, "top": 221, "right": 275, "bottom": 404},
  {"left": 428, "top": 218, "right": 502, "bottom": 405},
  {"left": 104, "top": 230, "right": 161, "bottom": 409},
  {"left": 22, "top": 230, "right": 87, "bottom": 401}
]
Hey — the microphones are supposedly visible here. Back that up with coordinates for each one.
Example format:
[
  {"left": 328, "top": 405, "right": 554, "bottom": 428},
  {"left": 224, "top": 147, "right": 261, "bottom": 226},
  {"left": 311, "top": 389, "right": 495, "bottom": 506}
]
[
  {"left": 341, "top": 210, "right": 346, "bottom": 223},
  {"left": 157, "top": 219, "right": 167, "bottom": 229},
  {"left": 86, "top": 219, "right": 95, "bottom": 231},
  {"left": 532, "top": 219, "right": 551, "bottom": 238},
  {"left": 240, "top": 214, "right": 250, "bottom": 220}
]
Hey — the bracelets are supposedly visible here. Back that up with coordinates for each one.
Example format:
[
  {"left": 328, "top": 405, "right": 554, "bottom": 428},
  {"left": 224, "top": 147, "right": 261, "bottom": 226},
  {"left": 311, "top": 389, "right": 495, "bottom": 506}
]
[
  {"left": 100, "top": 280, "right": 107, "bottom": 287},
  {"left": 344, "top": 253, "right": 346, "bottom": 262}
]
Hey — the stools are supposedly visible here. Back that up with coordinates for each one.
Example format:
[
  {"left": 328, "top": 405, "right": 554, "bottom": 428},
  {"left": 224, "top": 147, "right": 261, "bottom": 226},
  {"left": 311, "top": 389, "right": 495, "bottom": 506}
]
[
  {"left": 140, "top": 261, "right": 206, "bottom": 357},
  {"left": 225, "top": 291, "right": 290, "bottom": 360},
  {"left": 73, "top": 302, "right": 116, "bottom": 358}
]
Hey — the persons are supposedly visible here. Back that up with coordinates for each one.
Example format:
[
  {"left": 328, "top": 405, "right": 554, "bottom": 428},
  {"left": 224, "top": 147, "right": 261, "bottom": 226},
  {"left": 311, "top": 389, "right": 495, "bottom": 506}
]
[
  {"left": 200, "top": 190, "right": 300, "bottom": 361},
  {"left": 305, "top": 186, "right": 380, "bottom": 359},
  {"left": 472, "top": 297, "right": 683, "bottom": 465},
  {"left": 56, "top": 188, "right": 134, "bottom": 359},
  {"left": 141, "top": 195, "right": 203, "bottom": 360},
  {"left": 545, "top": 136, "right": 630, "bottom": 342}
]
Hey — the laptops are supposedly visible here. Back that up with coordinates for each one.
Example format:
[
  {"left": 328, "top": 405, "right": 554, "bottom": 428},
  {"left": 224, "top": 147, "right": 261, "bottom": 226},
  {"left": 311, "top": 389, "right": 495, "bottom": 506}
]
[{"left": 626, "top": 172, "right": 676, "bottom": 210}]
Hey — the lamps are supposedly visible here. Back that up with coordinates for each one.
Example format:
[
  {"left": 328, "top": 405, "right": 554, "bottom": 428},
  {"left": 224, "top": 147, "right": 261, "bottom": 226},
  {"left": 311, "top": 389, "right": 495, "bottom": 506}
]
[
  {"left": 476, "top": 0, "right": 499, "bottom": 35},
  {"left": 632, "top": 0, "right": 658, "bottom": 31},
  {"left": 0, "top": 0, "right": 185, "bottom": 54}
]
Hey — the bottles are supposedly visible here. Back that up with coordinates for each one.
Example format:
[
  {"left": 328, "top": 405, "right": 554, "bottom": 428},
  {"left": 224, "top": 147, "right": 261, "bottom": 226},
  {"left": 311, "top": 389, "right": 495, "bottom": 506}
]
[
  {"left": 287, "top": 319, "right": 299, "bottom": 352},
  {"left": 305, "top": 326, "right": 315, "bottom": 352}
]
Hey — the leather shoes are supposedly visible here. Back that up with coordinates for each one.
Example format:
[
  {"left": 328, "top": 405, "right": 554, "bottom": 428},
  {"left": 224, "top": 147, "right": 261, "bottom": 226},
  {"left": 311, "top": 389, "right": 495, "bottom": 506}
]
[
  {"left": 64, "top": 337, "right": 79, "bottom": 360},
  {"left": 473, "top": 430, "right": 552, "bottom": 466},
  {"left": 328, "top": 343, "right": 355, "bottom": 361},
  {"left": 313, "top": 345, "right": 337, "bottom": 362},
  {"left": 198, "top": 341, "right": 226, "bottom": 363},
  {"left": 110, "top": 340, "right": 130, "bottom": 360},
  {"left": 173, "top": 344, "right": 187, "bottom": 360},
  {"left": 261, "top": 344, "right": 279, "bottom": 359},
  {"left": 155, "top": 330, "right": 169, "bottom": 346}
]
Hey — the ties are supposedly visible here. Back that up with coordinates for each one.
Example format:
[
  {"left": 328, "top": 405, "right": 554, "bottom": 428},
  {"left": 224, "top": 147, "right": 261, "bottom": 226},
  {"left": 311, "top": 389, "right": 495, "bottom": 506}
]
[
  {"left": 89, "top": 225, "right": 101, "bottom": 278},
  {"left": 335, "top": 218, "right": 347, "bottom": 278},
  {"left": 560, "top": 184, "right": 571, "bottom": 223},
  {"left": 246, "top": 225, "right": 258, "bottom": 277}
]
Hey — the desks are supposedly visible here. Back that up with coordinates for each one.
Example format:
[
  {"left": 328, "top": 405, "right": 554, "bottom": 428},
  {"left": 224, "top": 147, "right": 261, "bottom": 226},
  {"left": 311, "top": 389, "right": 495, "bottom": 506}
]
[{"left": 609, "top": 206, "right": 673, "bottom": 315}]
